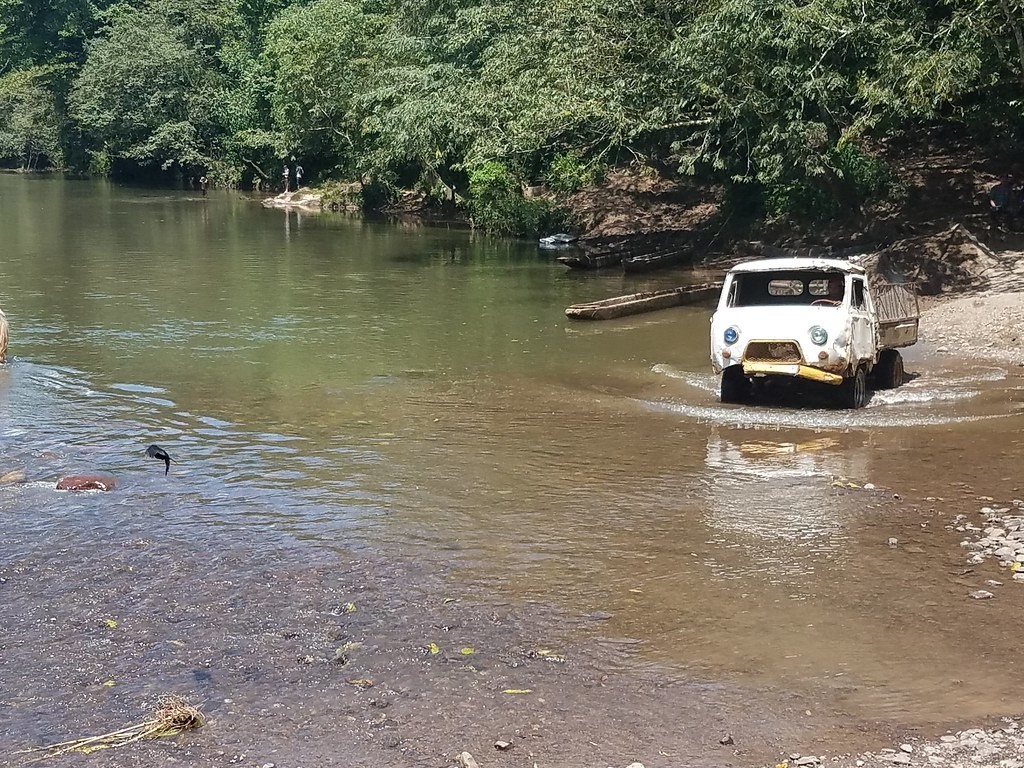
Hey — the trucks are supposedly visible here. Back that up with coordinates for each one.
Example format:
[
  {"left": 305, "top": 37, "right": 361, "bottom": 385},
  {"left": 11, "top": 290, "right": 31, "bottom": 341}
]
[{"left": 708, "top": 257, "right": 922, "bottom": 409}]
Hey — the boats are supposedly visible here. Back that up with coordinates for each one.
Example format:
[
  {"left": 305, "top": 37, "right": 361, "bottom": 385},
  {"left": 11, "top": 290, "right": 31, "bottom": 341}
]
[
  {"left": 540, "top": 233, "right": 578, "bottom": 244},
  {"left": 555, "top": 242, "right": 668, "bottom": 270},
  {"left": 564, "top": 280, "right": 723, "bottom": 320},
  {"left": 620, "top": 246, "right": 693, "bottom": 273}
]
